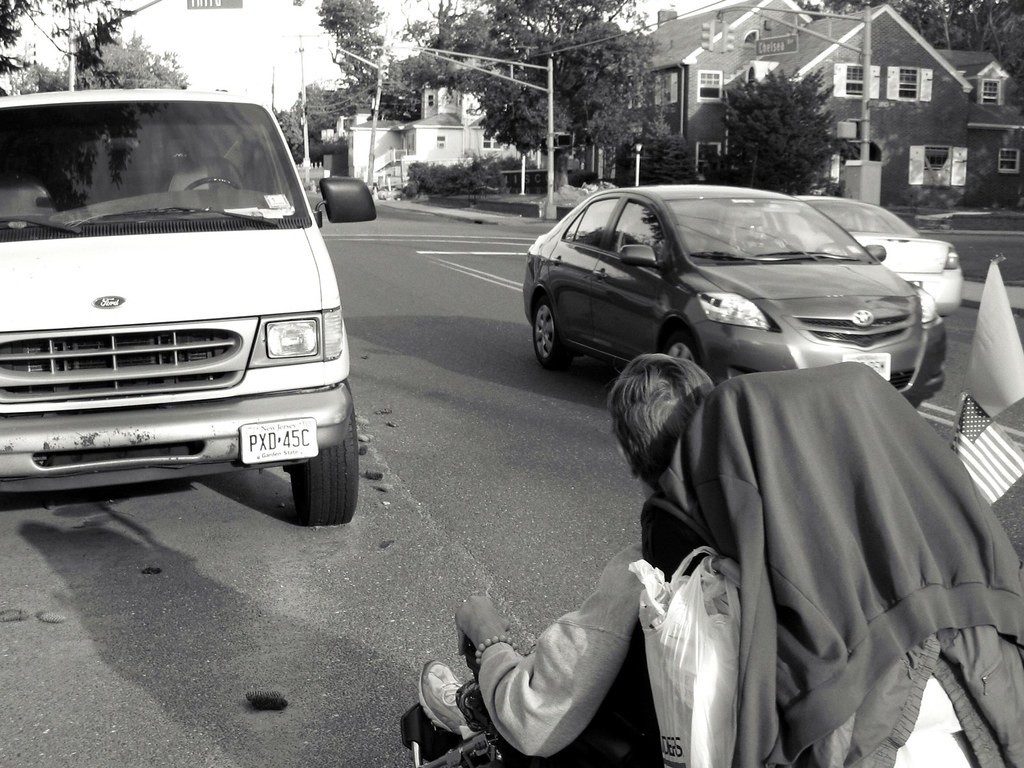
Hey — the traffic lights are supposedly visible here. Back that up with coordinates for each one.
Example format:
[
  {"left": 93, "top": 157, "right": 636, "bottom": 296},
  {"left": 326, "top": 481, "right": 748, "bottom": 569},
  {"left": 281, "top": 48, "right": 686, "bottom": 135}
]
[
  {"left": 378, "top": 55, "right": 390, "bottom": 80},
  {"left": 700, "top": 19, "right": 715, "bottom": 52},
  {"left": 721, "top": 21, "right": 735, "bottom": 54}
]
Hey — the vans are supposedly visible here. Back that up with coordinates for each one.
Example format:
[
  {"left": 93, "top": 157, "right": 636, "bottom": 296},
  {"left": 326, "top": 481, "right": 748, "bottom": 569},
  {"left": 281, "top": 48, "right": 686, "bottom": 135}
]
[{"left": 0, "top": 89, "right": 380, "bottom": 526}]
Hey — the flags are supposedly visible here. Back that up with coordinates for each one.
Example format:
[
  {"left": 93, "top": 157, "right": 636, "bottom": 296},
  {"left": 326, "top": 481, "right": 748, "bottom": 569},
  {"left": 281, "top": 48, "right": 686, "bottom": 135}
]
[{"left": 949, "top": 390, "right": 1024, "bottom": 504}]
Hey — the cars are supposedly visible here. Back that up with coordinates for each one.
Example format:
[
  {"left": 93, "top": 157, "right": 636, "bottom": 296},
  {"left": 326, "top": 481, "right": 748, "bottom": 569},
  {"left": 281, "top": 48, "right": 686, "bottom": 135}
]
[
  {"left": 781, "top": 194, "right": 964, "bottom": 317},
  {"left": 520, "top": 182, "right": 949, "bottom": 410}
]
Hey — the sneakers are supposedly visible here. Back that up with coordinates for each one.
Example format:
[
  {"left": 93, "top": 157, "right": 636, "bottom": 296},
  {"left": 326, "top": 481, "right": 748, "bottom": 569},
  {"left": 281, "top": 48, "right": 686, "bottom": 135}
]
[{"left": 418, "top": 659, "right": 502, "bottom": 768}]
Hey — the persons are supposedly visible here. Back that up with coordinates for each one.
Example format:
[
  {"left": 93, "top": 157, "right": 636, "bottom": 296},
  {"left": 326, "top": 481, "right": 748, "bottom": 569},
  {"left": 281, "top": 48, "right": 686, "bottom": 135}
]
[{"left": 418, "top": 353, "right": 716, "bottom": 767}]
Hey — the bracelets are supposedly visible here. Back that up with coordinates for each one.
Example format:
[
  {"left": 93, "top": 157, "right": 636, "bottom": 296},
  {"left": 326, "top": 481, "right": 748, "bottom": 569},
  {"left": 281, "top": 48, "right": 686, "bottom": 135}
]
[{"left": 475, "top": 634, "right": 518, "bottom": 663}]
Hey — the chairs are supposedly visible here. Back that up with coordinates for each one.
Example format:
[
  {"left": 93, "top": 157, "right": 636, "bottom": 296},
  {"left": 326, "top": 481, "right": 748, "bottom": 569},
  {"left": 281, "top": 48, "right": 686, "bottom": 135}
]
[
  {"left": 169, "top": 156, "right": 242, "bottom": 192},
  {"left": 0, "top": 179, "right": 58, "bottom": 216}
]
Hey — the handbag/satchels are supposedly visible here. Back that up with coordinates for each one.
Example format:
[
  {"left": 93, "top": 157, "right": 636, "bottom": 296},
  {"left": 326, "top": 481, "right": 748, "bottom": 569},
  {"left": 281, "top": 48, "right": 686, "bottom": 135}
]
[{"left": 638, "top": 544, "right": 742, "bottom": 768}]
